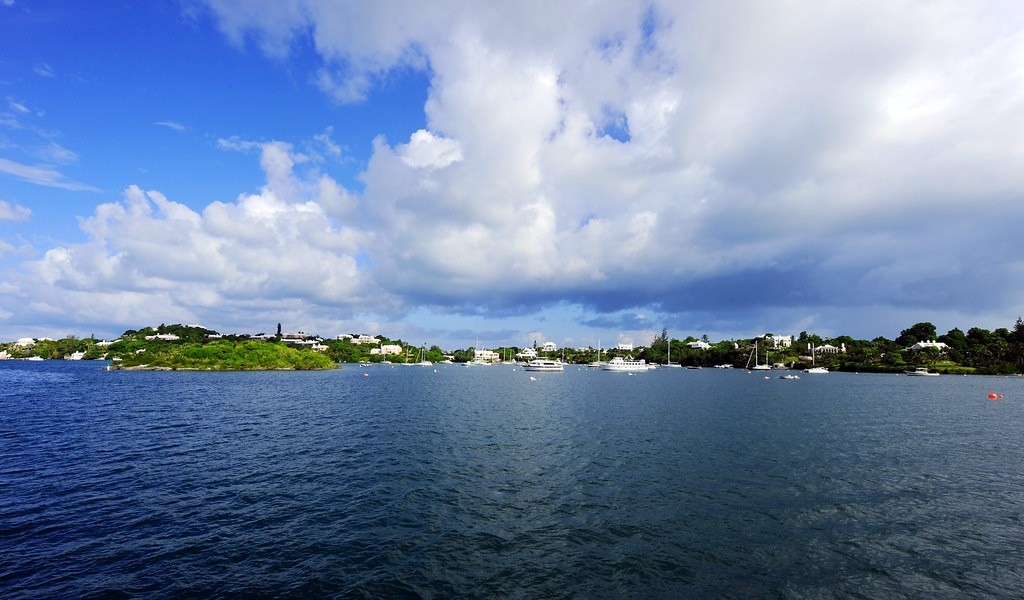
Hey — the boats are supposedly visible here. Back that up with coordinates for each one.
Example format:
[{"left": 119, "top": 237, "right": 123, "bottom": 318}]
[
  {"left": 599, "top": 357, "right": 648, "bottom": 371},
  {"left": 521, "top": 356, "right": 564, "bottom": 371},
  {"left": 780, "top": 375, "right": 800, "bottom": 379},
  {"left": 907, "top": 368, "right": 940, "bottom": 376},
  {"left": 753, "top": 364, "right": 771, "bottom": 369},
  {"left": 803, "top": 367, "right": 830, "bottom": 374}
]
